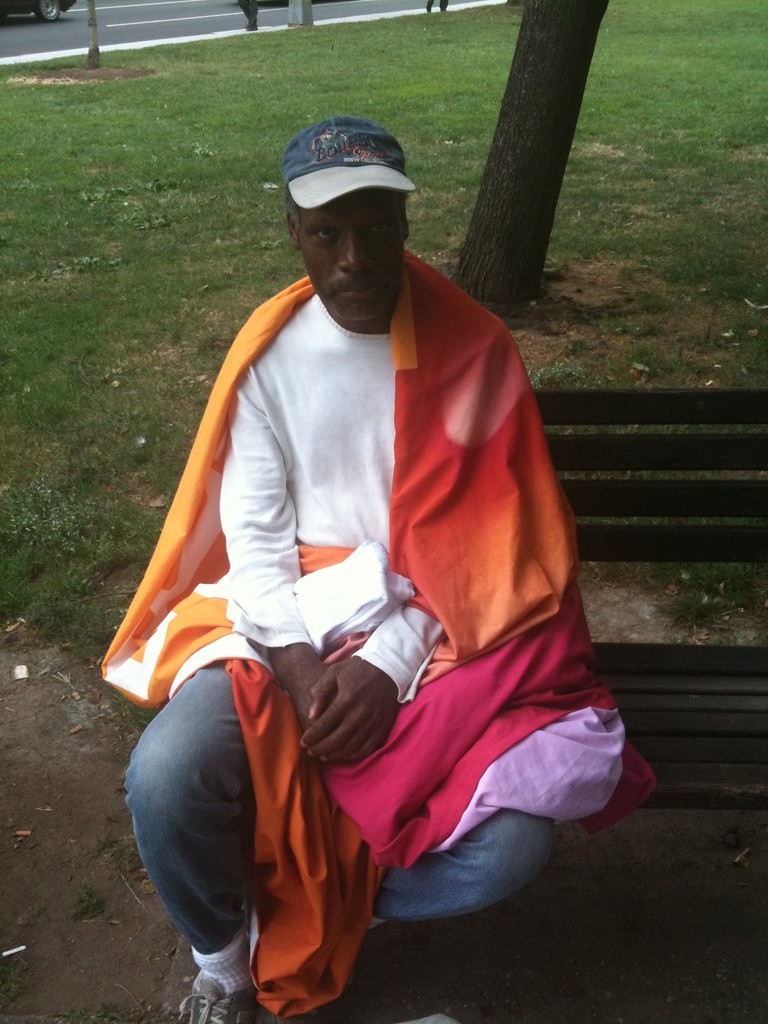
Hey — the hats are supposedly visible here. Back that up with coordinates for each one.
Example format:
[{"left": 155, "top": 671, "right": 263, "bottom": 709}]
[{"left": 282, "top": 114, "right": 416, "bottom": 209}]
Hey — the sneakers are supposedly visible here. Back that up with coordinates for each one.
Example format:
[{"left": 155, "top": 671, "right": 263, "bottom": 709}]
[
  {"left": 180, "top": 970, "right": 259, "bottom": 1023},
  {"left": 256, "top": 1003, "right": 321, "bottom": 1024}
]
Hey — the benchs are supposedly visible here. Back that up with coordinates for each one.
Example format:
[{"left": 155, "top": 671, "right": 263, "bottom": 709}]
[{"left": 524, "top": 391, "right": 768, "bottom": 814}]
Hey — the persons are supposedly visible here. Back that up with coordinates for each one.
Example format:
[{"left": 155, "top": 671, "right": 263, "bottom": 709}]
[
  {"left": 101, "top": 118, "right": 656, "bottom": 1024},
  {"left": 426, "top": 0, "right": 448, "bottom": 12},
  {"left": 238, "top": 0, "right": 258, "bottom": 31}
]
